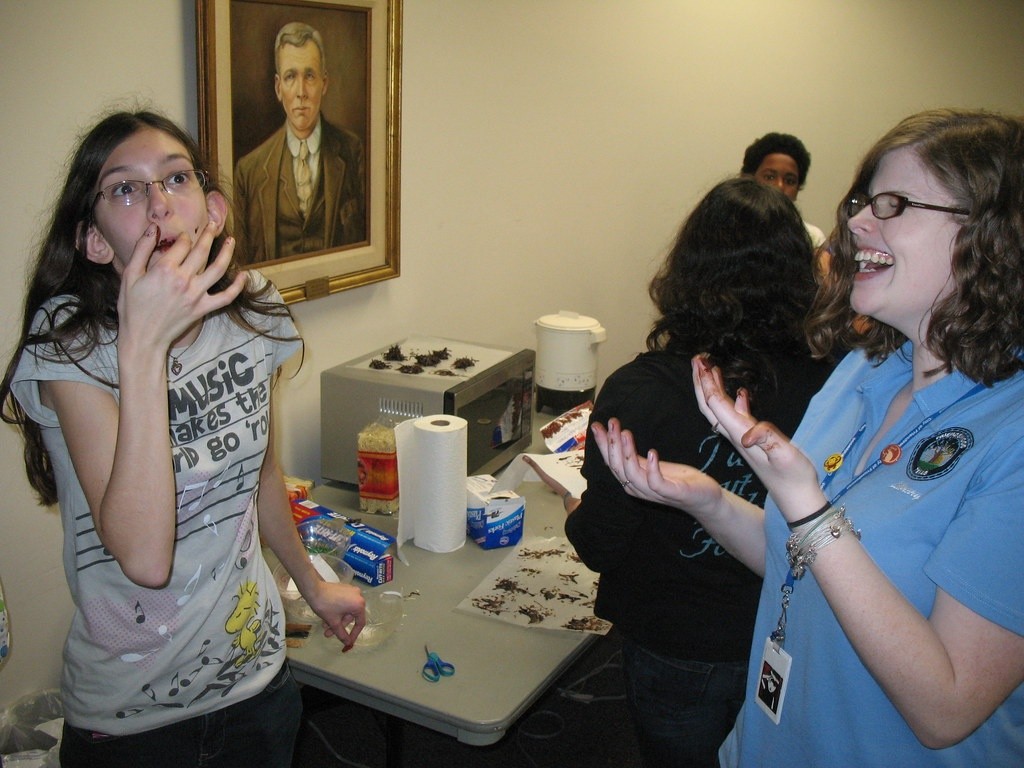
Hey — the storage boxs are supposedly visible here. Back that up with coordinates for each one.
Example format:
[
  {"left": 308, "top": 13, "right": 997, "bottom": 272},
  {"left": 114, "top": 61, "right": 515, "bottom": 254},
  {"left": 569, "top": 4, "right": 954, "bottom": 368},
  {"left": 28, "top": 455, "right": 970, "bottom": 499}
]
[
  {"left": 466, "top": 475, "right": 525, "bottom": 551},
  {"left": 290, "top": 500, "right": 396, "bottom": 587}
]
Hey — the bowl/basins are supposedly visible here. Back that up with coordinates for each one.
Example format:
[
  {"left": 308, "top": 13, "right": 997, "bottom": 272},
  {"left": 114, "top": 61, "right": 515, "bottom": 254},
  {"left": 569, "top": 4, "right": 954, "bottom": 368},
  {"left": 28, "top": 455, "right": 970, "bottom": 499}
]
[
  {"left": 273, "top": 554, "right": 352, "bottom": 623},
  {"left": 346, "top": 592, "right": 403, "bottom": 646},
  {"left": 296, "top": 520, "right": 350, "bottom": 560}
]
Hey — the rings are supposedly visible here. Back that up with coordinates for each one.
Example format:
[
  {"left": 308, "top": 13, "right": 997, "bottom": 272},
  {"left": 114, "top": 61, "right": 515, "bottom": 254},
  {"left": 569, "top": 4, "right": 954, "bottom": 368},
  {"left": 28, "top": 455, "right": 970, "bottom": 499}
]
[
  {"left": 710, "top": 421, "right": 720, "bottom": 435},
  {"left": 620, "top": 481, "right": 626, "bottom": 488},
  {"left": 626, "top": 480, "right": 630, "bottom": 485}
]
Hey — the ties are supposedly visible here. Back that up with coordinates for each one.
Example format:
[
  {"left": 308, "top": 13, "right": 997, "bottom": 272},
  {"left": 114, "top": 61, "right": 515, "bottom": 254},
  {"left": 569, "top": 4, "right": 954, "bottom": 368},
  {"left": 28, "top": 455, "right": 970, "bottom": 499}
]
[{"left": 294, "top": 139, "right": 313, "bottom": 224}]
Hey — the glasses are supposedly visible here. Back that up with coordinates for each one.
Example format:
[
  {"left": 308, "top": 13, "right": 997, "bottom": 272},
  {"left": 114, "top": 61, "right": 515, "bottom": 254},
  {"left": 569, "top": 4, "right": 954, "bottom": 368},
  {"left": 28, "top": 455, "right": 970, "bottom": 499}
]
[
  {"left": 85, "top": 169, "right": 209, "bottom": 213},
  {"left": 847, "top": 191, "right": 970, "bottom": 220}
]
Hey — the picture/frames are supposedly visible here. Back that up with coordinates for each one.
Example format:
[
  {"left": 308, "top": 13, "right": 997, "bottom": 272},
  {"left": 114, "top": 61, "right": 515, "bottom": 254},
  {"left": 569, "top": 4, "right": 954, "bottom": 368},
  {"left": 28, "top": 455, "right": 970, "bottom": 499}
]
[{"left": 194, "top": 0, "right": 402, "bottom": 305}]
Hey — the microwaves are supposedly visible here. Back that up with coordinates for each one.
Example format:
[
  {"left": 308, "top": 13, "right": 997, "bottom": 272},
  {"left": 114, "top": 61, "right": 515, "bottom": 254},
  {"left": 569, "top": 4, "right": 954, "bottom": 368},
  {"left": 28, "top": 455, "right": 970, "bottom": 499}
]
[{"left": 320, "top": 338, "right": 534, "bottom": 483}]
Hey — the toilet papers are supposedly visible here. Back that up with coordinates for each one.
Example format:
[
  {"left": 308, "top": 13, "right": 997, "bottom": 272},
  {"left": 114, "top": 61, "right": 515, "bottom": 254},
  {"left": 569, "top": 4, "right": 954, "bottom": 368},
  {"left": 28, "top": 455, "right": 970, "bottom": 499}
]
[{"left": 394, "top": 415, "right": 469, "bottom": 553}]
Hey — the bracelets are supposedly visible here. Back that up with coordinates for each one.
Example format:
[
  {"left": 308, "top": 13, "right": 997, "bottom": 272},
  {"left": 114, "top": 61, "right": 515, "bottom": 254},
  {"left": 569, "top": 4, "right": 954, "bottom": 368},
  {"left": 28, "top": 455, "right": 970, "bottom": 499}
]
[
  {"left": 785, "top": 501, "right": 862, "bottom": 581},
  {"left": 562, "top": 492, "right": 571, "bottom": 511}
]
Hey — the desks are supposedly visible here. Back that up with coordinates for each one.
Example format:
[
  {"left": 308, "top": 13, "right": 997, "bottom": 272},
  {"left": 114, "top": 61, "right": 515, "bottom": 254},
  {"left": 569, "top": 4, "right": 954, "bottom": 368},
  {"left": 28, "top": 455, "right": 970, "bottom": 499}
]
[{"left": 263, "top": 392, "right": 614, "bottom": 768}]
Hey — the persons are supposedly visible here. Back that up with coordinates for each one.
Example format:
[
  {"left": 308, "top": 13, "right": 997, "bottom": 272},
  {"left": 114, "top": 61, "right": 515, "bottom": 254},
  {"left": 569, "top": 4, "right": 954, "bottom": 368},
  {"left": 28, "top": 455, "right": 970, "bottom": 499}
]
[
  {"left": 591, "top": 109, "right": 1024, "bottom": 768},
  {"left": 0, "top": 112, "right": 368, "bottom": 768},
  {"left": 520, "top": 131, "right": 875, "bottom": 768}
]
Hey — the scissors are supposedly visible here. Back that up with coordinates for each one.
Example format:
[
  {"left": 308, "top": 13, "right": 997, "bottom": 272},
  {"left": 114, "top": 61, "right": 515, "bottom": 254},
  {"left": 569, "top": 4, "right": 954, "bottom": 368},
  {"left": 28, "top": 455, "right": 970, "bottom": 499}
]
[{"left": 421, "top": 644, "right": 456, "bottom": 682}]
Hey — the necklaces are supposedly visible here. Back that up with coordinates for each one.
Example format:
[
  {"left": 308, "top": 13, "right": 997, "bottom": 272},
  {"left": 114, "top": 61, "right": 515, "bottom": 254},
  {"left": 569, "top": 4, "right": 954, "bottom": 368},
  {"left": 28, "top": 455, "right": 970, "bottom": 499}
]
[{"left": 167, "top": 315, "right": 206, "bottom": 375}]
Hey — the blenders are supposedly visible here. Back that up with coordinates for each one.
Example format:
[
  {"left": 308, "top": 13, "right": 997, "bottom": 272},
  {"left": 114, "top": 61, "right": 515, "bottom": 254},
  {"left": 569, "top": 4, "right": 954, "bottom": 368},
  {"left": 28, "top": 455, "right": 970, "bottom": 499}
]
[{"left": 535, "top": 312, "right": 605, "bottom": 415}]
